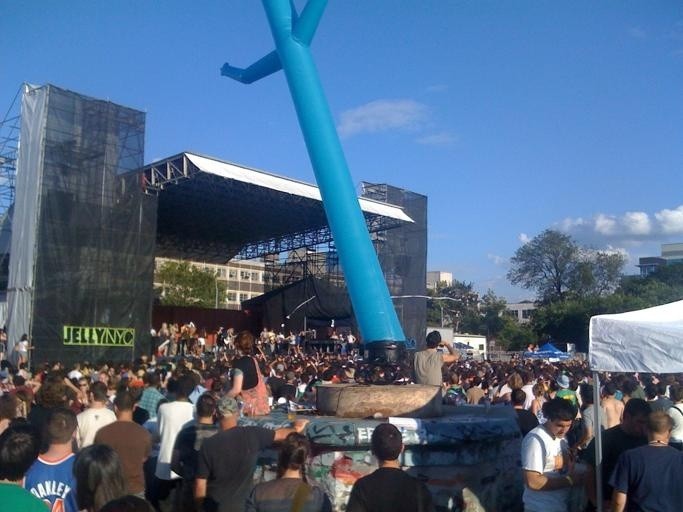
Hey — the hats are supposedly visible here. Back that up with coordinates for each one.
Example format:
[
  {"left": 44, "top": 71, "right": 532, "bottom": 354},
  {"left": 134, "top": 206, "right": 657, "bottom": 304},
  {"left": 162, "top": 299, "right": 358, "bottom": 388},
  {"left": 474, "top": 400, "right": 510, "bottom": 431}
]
[
  {"left": 214, "top": 396, "right": 238, "bottom": 414},
  {"left": 556, "top": 374, "right": 569, "bottom": 388}
]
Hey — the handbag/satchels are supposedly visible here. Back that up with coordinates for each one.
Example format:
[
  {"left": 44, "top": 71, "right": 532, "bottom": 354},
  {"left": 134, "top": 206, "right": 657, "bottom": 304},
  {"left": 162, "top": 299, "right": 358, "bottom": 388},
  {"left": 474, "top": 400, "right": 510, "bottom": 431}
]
[{"left": 237, "top": 375, "right": 269, "bottom": 415}]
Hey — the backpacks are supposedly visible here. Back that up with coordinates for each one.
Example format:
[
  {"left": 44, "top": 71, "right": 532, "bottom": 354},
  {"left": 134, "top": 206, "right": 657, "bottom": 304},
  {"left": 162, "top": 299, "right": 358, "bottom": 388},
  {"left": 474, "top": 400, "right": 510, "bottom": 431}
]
[{"left": 449, "top": 388, "right": 467, "bottom": 406}]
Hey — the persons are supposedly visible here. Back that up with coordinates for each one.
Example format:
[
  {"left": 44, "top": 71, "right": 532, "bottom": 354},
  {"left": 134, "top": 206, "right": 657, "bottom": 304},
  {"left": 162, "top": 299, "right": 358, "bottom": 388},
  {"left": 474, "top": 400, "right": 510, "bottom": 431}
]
[{"left": 2, "top": 314, "right": 683, "bottom": 511}]
